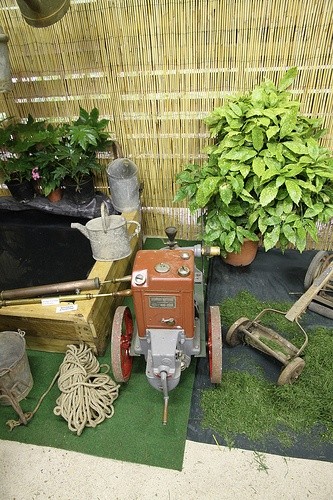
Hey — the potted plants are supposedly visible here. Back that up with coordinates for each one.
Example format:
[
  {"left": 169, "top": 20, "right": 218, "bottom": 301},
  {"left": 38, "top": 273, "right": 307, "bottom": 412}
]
[
  {"left": 0, "top": 107, "right": 114, "bottom": 205},
  {"left": 175, "top": 63, "right": 333, "bottom": 267}
]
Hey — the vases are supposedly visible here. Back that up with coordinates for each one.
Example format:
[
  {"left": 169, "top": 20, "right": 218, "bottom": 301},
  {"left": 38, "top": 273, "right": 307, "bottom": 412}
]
[{"left": 48, "top": 188, "right": 61, "bottom": 202}]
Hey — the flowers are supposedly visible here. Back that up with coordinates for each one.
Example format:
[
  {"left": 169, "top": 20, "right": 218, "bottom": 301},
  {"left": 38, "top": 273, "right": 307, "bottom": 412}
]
[{"left": 32, "top": 163, "right": 57, "bottom": 196}]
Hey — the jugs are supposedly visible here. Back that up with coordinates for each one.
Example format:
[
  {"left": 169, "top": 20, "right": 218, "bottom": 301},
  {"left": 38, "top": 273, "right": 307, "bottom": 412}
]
[{"left": 71, "top": 203, "right": 143, "bottom": 261}]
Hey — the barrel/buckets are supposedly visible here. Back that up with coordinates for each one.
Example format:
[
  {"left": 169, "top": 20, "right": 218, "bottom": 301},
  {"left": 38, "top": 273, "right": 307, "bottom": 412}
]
[
  {"left": 1, "top": 327, "right": 34, "bottom": 404},
  {"left": 108, "top": 159, "right": 143, "bottom": 212}
]
